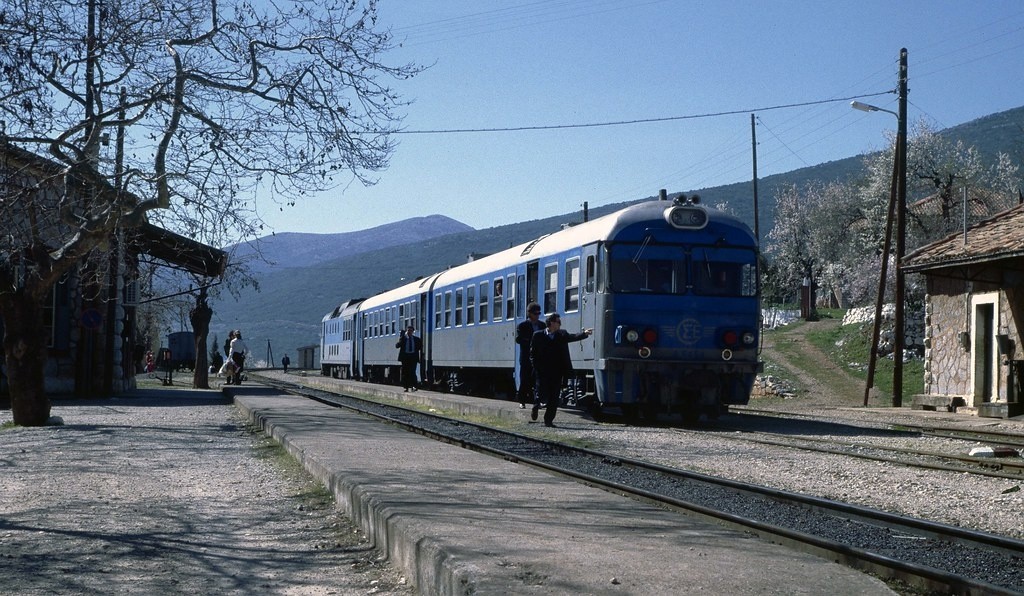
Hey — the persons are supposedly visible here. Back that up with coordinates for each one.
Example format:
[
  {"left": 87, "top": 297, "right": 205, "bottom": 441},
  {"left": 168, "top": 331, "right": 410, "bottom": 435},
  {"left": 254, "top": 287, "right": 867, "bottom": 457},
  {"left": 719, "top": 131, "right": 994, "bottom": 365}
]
[
  {"left": 212, "top": 351, "right": 223, "bottom": 377},
  {"left": 529, "top": 313, "right": 595, "bottom": 427},
  {"left": 396, "top": 326, "right": 423, "bottom": 392},
  {"left": 224, "top": 330, "right": 248, "bottom": 385},
  {"left": 282, "top": 354, "right": 290, "bottom": 373},
  {"left": 496, "top": 282, "right": 502, "bottom": 295},
  {"left": 515, "top": 302, "right": 547, "bottom": 409},
  {"left": 146, "top": 350, "right": 155, "bottom": 372}
]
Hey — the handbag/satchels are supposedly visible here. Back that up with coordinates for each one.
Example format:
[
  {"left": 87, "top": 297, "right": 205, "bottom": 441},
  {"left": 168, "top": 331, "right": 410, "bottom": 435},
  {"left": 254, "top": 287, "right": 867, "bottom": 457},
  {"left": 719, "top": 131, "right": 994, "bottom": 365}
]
[{"left": 219, "top": 358, "right": 241, "bottom": 377}]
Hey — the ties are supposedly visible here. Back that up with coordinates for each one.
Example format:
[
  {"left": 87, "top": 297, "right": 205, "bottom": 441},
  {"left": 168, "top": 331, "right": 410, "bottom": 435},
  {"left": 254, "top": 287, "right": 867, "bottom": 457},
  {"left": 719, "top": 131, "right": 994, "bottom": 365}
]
[{"left": 409, "top": 337, "right": 412, "bottom": 352}]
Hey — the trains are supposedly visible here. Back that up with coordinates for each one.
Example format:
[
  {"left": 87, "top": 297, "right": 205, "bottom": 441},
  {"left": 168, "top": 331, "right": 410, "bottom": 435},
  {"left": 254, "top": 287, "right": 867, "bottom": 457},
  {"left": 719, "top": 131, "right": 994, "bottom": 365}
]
[{"left": 320, "top": 198, "right": 766, "bottom": 417}]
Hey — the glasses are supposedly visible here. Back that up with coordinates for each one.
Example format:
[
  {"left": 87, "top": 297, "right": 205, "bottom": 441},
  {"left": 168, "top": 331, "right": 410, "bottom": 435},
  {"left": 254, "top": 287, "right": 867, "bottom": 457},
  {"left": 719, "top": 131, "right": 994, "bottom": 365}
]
[
  {"left": 554, "top": 321, "right": 561, "bottom": 324},
  {"left": 531, "top": 311, "right": 541, "bottom": 315}
]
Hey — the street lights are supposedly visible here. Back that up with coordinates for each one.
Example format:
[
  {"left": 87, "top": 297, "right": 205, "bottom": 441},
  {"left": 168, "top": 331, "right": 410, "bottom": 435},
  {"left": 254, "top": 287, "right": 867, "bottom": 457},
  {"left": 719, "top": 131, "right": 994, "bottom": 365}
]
[{"left": 851, "top": 99, "right": 903, "bottom": 407}]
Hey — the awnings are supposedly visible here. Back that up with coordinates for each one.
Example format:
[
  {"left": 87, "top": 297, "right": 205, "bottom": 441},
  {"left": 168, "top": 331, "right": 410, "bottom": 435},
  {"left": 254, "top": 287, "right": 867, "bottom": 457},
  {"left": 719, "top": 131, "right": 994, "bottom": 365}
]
[{"left": 135, "top": 223, "right": 228, "bottom": 303}]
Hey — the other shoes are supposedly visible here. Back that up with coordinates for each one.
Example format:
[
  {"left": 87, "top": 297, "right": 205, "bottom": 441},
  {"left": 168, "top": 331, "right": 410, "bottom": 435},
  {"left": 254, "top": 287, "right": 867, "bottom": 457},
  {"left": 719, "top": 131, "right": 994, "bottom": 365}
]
[
  {"left": 531, "top": 410, "right": 538, "bottom": 420},
  {"left": 544, "top": 418, "right": 556, "bottom": 427},
  {"left": 412, "top": 387, "right": 417, "bottom": 391},
  {"left": 519, "top": 403, "right": 525, "bottom": 408},
  {"left": 404, "top": 387, "right": 408, "bottom": 392}
]
